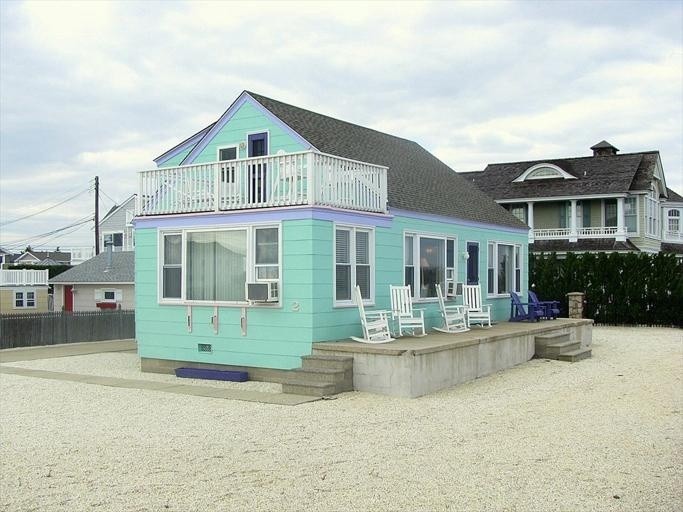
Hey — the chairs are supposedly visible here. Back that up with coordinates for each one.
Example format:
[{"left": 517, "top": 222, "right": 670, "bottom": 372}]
[
  {"left": 508, "top": 289, "right": 561, "bottom": 323},
  {"left": 431, "top": 283, "right": 493, "bottom": 335},
  {"left": 349, "top": 282, "right": 428, "bottom": 345},
  {"left": 268, "top": 148, "right": 363, "bottom": 206},
  {"left": 176, "top": 176, "right": 240, "bottom": 208}
]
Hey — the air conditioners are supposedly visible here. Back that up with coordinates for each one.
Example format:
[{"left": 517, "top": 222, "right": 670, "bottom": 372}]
[{"left": 244, "top": 279, "right": 278, "bottom": 304}]
